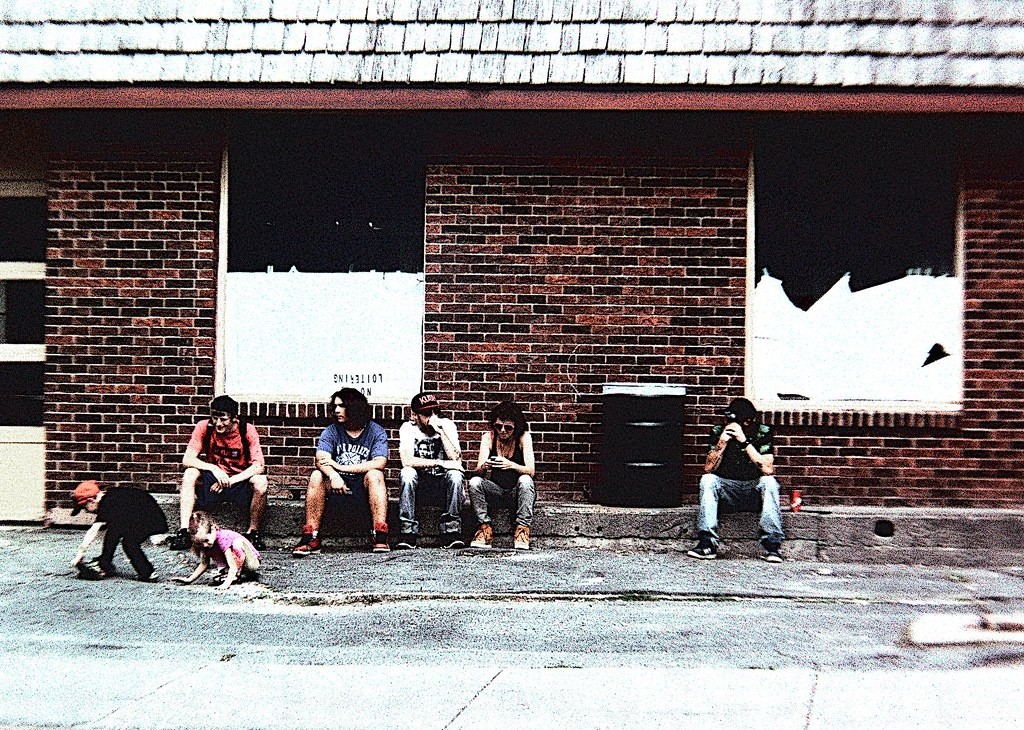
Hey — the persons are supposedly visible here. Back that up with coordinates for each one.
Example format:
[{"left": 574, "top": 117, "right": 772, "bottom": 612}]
[
  {"left": 468, "top": 402, "right": 537, "bottom": 549},
  {"left": 292, "top": 388, "right": 390, "bottom": 555},
  {"left": 395, "top": 392, "right": 464, "bottom": 548},
  {"left": 171, "top": 395, "right": 268, "bottom": 552},
  {"left": 686, "top": 397, "right": 784, "bottom": 563},
  {"left": 69, "top": 481, "right": 168, "bottom": 581},
  {"left": 171, "top": 512, "right": 261, "bottom": 590}
]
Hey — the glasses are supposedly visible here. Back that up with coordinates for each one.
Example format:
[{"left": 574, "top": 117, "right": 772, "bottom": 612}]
[
  {"left": 494, "top": 423, "right": 516, "bottom": 433},
  {"left": 414, "top": 408, "right": 442, "bottom": 417}
]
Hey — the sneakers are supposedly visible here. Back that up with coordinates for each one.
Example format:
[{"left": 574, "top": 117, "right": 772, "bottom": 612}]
[
  {"left": 170, "top": 528, "right": 193, "bottom": 550},
  {"left": 686, "top": 540, "right": 718, "bottom": 560},
  {"left": 440, "top": 532, "right": 465, "bottom": 547},
  {"left": 370, "top": 522, "right": 390, "bottom": 552},
  {"left": 292, "top": 525, "right": 321, "bottom": 556},
  {"left": 242, "top": 529, "right": 267, "bottom": 551},
  {"left": 758, "top": 544, "right": 783, "bottom": 563},
  {"left": 513, "top": 525, "right": 531, "bottom": 549},
  {"left": 469, "top": 524, "right": 494, "bottom": 549},
  {"left": 393, "top": 535, "right": 419, "bottom": 549}
]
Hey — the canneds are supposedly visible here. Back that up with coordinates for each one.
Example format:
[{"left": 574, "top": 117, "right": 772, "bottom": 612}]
[{"left": 790, "top": 489, "right": 800, "bottom": 512}]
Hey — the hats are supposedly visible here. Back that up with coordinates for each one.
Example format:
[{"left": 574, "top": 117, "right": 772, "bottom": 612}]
[
  {"left": 726, "top": 399, "right": 755, "bottom": 424},
  {"left": 211, "top": 394, "right": 239, "bottom": 416},
  {"left": 410, "top": 391, "right": 445, "bottom": 410},
  {"left": 70, "top": 480, "right": 100, "bottom": 517}
]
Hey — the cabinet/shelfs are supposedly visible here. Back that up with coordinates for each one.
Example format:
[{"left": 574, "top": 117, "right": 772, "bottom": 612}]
[{"left": 599, "top": 382, "right": 686, "bottom": 510}]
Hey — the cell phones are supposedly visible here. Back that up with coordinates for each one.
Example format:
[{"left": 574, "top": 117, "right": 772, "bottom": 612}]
[{"left": 490, "top": 456, "right": 502, "bottom": 465}]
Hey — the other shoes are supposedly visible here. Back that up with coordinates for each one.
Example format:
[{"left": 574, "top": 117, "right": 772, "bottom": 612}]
[
  {"left": 135, "top": 570, "right": 159, "bottom": 582},
  {"left": 78, "top": 562, "right": 104, "bottom": 573},
  {"left": 213, "top": 573, "right": 238, "bottom": 585}
]
